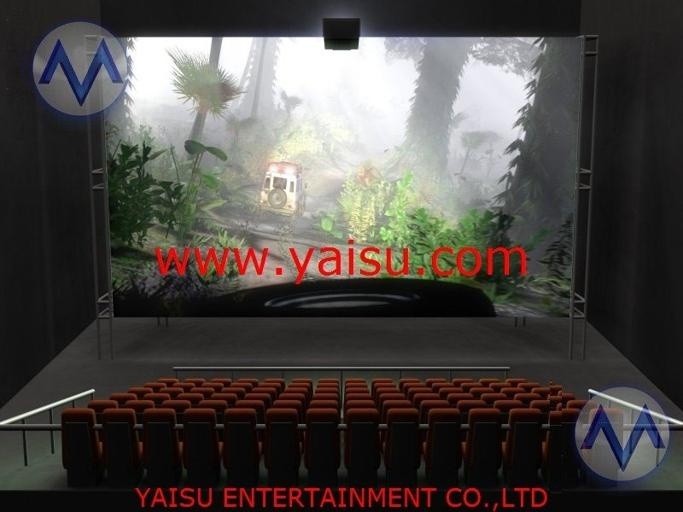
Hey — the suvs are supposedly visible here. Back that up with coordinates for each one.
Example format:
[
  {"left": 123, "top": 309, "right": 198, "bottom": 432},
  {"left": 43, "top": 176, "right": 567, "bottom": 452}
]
[{"left": 256, "top": 159, "right": 306, "bottom": 219}]
